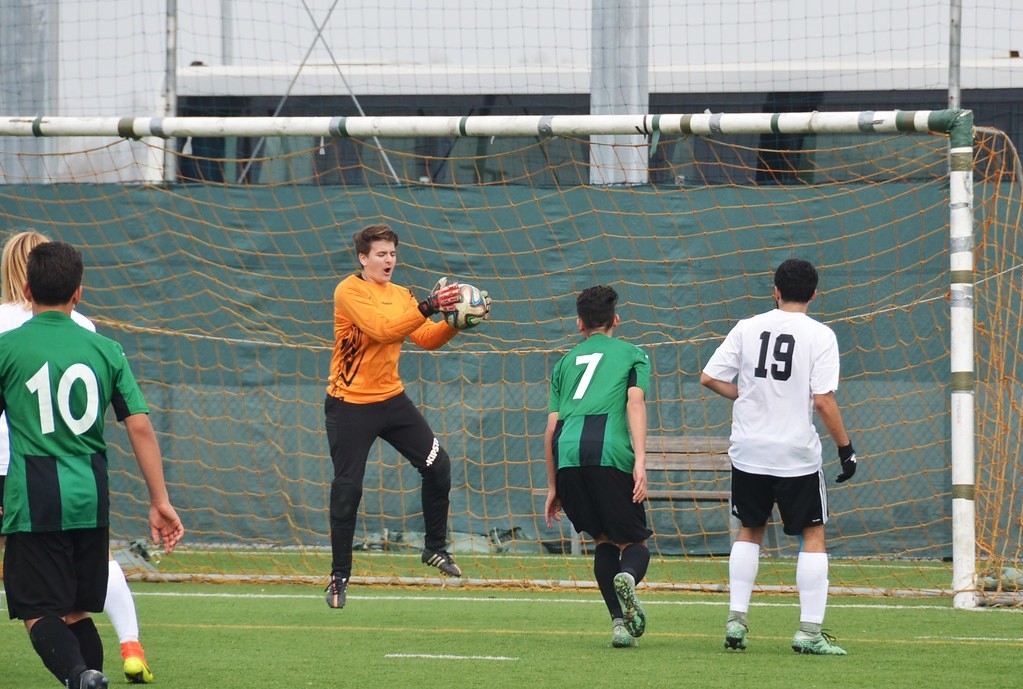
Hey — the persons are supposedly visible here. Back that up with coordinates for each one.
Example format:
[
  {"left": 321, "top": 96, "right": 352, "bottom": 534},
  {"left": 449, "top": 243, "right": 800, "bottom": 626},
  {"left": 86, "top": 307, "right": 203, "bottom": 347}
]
[
  {"left": 543, "top": 286, "right": 654, "bottom": 651},
  {"left": 0, "top": 228, "right": 154, "bottom": 683},
  {"left": 324, "top": 225, "right": 492, "bottom": 611},
  {"left": 700, "top": 260, "right": 857, "bottom": 657},
  {"left": 1, "top": 241, "right": 186, "bottom": 689}
]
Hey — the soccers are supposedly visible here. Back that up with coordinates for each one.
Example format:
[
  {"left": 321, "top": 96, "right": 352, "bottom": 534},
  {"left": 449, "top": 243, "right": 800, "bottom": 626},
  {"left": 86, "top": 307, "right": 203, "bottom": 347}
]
[{"left": 441, "top": 283, "right": 486, "bottom": 331}]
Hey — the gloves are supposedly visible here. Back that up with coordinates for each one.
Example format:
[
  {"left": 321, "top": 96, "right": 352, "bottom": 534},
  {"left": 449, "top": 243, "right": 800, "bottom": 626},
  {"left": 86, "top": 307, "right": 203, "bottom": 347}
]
[
  {"left": 832, "top": 443, "right": 858, "bottom": 484},
  {"left": 479, "top": 289, "right": 492, "bottom": 322},
  {"left": 416, "top": 277, "right": 463, "bottom": 318}
]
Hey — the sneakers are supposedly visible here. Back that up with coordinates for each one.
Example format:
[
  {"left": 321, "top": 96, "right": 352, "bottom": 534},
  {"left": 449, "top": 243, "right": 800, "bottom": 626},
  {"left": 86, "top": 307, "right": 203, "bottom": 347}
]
[
  {"left": 326, "top": 573, "right": 348, "bottom": 610},
  {"left": 609, "top": 619, "right": 639, "bottom": 648},
  {"left": 75, "top": 667, "right": 109, "bottom": 689},
  {"left": 722, "top": 618, "right": 748, "bottom": 654},
  {"left": 612, "top": 571, "right": 648, "bottom": 639},
  {"left": 420, "top": 549, "right": 463, "bottom": 577},
  {"left": 791, "top": 629, "right": 848, "bottom": 656},
  {"left": 121, "top": 656, "right": 154, "bottom": 684}
]
